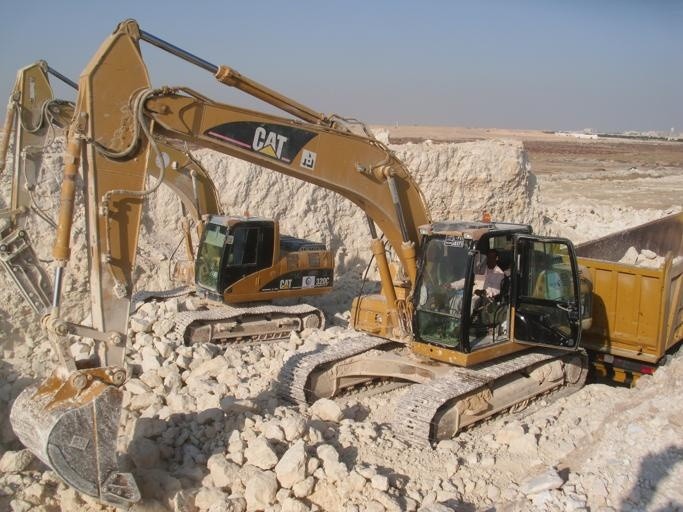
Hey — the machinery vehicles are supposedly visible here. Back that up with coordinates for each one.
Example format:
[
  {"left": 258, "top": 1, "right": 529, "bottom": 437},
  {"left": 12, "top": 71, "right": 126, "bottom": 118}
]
[
  {"left": 9, "top": 17, "right": 590, "bottom": 504},
  {"left": 0, "top": 56, "right": 339, "bottom": 351}
]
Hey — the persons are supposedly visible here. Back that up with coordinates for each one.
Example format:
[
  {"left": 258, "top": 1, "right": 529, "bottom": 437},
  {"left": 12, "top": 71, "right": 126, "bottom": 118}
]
[{"left": 442, "top": 252, "right": 505, "bottom": 337}]
